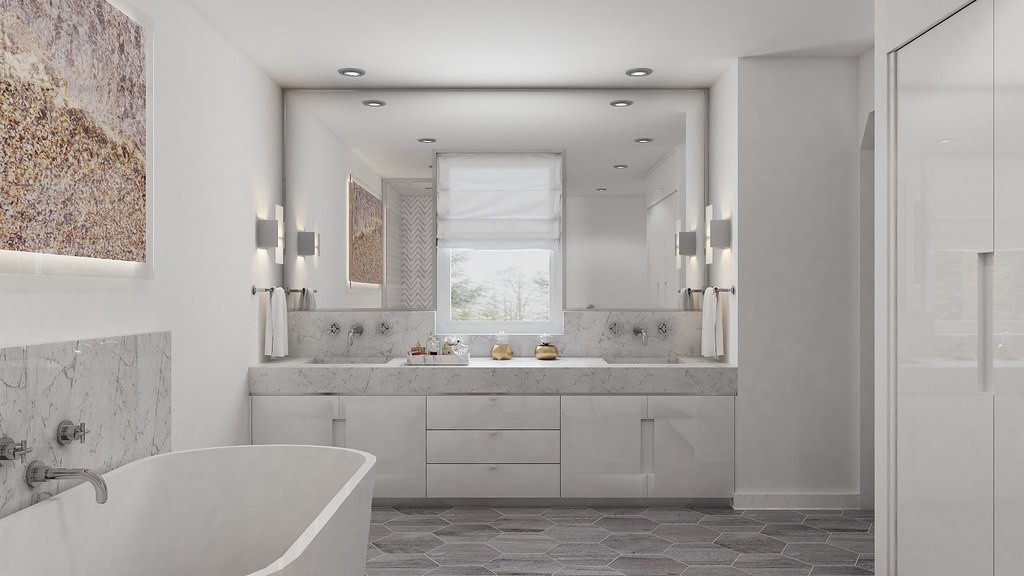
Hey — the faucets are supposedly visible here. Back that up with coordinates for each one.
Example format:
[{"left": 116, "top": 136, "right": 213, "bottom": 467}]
[
  {"left": 632, "top": 324, "right": 649, "bottom": 347},
  {"left": 346, "top": 322, "right": 364, "bottom": 349},
  {"left": 25, "top": 458, "right": 110, "bottom": 506}
]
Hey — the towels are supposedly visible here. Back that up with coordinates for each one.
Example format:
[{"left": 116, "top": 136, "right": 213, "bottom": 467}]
[
  {"left": 263, "top": 285, "right": 290, "bottom": 360},
  {"left": 675, "top": 287, "right": 699, "bottom": 312},
  {"left": 700, "top": 286, "right": 725, "bottom": 359},
  {"left": 296, "top": 288, "right": 317, "bottom": 309}
]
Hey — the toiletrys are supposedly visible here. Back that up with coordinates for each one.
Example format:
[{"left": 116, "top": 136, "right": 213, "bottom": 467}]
[{"left": 407, "top": 334, "right": 467, "bottom": 357}]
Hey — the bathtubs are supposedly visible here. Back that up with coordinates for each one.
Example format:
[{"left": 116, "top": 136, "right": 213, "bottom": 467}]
[{"left": 0, "top": 442, "right": 378, "bottom": 576}]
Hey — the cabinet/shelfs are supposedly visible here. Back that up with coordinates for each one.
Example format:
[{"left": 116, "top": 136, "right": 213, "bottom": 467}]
[
  {"left": 561, "top": 395, "right": 732, "bottom": 509},
  {"left": 886, "top": 0, "right": 1024, "bottom": 574},
  {"left": 251, "top": 395, "right": 426, "bottom": 507},
  {"left": 427, "top": 396, "right": 560, "bottom": 507}
]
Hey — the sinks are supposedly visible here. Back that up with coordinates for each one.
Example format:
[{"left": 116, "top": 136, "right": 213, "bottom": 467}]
[
  {"left": 601, "top": 354, "right": 691, "bottom": 367},
  {"left": 304, "top": 353, "right": 394, "bottom": 366}
]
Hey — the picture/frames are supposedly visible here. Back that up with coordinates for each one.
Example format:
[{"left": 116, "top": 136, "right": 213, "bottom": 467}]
[{"left": 0, "top": 0, "right": 155, "bottom": 281}]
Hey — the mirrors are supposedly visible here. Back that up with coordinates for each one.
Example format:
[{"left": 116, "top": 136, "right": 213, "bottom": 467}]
[{"left": 281, "top": 86, "right": 708, "bottom": 313}]
